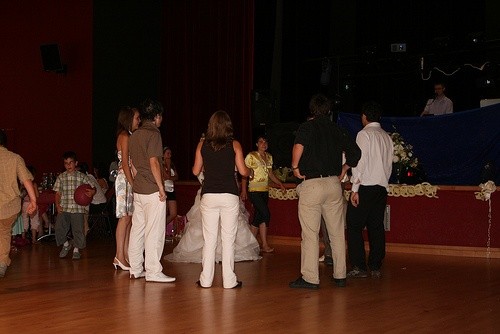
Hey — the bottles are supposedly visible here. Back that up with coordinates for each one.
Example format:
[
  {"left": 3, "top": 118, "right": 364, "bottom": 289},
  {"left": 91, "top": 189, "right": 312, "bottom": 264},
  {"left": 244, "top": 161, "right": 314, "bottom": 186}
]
[
  {"left": 42, "top": 174, "right": 47, "bottom": 187},
  {"left": 49, "top": 172, "right": 54, "bottom": 185}
]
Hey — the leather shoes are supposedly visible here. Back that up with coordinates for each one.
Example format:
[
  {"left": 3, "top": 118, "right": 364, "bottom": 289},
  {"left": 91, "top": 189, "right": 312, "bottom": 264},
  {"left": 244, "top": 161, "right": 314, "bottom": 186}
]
[
  {"left": 335, "top": 278, "right": 347, "bottom": 286},
  {"left": 289, "top": 276, "right": 319, "bottom": 289}
]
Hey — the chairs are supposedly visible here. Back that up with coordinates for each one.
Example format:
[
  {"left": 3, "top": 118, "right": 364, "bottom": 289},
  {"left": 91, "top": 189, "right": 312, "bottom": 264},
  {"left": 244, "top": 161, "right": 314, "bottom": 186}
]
[{"left": 86, "top": 187, "right": 115, "bottom": 237}]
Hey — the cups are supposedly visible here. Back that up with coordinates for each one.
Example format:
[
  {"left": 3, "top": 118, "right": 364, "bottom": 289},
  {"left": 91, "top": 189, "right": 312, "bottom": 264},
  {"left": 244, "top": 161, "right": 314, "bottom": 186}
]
[{"left": 39, "top": 187, "right": 42, "bottom": 193}]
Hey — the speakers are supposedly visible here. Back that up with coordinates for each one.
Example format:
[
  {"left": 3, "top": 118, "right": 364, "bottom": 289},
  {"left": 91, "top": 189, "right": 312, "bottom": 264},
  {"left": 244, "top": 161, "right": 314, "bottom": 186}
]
[{"left": 41, "top": 44, "right": 63, "bottom": 72}]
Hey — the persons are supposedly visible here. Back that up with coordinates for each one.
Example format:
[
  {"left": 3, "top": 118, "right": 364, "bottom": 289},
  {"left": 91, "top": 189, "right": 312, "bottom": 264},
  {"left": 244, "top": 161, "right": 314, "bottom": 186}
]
[
  {"left": 129, "top": 101, "right": 177, "bottom": 283},
  {"left": 51, "top": 152, "right": 97, "bottom": 259},
  {"left": 0, "top": 130, "right": 38, "bottom": 278},
  {"left": 286, "top": 92, "right": 362, "bottom": 290},
  {"left": 113, "top": 105, "right": 142, "bottom": 272},
  {"left": 197, "top": 111, "right": 253, "bottom": 289},
  {"left": 162, "top": 138, "right": 265, "bottom": 263},
  {"left": 240, "top": 136, "right": 286, "bottom": 253},
  {"left": 350, "top": 103, "right": 394, "bottom": 279},
  {"left": 421, "top": 83, "right": 453, "bottom": 117},
  {"left": 11, "top": 172, "right": 50, "bottom": 247},
  {"left": 163, "top": 146, "right": 178, "bottom": 227},
  {"left": 71, "top": 167, "right": 110, "bottom": 249}
]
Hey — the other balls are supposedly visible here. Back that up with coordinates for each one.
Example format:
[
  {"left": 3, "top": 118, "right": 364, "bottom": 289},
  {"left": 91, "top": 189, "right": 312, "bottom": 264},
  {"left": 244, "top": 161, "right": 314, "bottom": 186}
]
[{"left": 74, "top": 184, "right": 93, "bottom": 206}]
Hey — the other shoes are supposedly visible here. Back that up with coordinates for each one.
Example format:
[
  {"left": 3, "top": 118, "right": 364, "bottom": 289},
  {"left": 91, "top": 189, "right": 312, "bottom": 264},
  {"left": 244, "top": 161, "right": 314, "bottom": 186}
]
[
  {"left": 146, "top": 271, "right": 175, "bottom": 283},
  {"left": 72, "top": 251, "right": 80, "bottom": 259},
  {"left": 59, "top": 241, "right": 72, "bottom": 258},
  {"left": 319, "top": 254, "right": 326, "bottom": 262},
  {"left": 129, "top": 269, "right": 145, "bottom": 278},
  {"left": 234, "top": 280, "right": 242, "bottom": 288},
  {"left": 196, "top": 280, "right": 201, "bottom": 287},
  {"left": 324, "top": 256, "right": 332, "bottom": 265},
  {"left": 370, "top": 270, "right": 381, "bottom": 279},
  {"left": 347, "top": 269, "right": 368, "bottom": 278},
  {"left": 0, "top": 266, "right": 8, "bottom": 278},
  {"left": 262, "top": 246, "right": 275, "bottom": 252}
]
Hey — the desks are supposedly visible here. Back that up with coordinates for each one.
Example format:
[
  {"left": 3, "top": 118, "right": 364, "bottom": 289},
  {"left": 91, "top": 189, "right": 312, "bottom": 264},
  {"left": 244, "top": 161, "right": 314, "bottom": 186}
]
[
  {"left": 339, "top": 102, "right": 500, "bottom": 188},
  {"left": 36, "top": 191, "right": 73, "bottom": 241}
]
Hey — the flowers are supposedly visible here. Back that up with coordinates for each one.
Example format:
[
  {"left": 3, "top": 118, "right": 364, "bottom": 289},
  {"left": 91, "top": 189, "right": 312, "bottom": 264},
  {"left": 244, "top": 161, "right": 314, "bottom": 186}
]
[{"left": 390, "top": 131, "right": 419, "bottom": 168}]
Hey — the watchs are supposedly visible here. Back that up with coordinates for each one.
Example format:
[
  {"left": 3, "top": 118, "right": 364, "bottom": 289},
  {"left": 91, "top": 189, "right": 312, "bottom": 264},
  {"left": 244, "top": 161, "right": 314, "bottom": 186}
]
[{"left": 291, "top": 166, "right": 300, "bottom": 171}]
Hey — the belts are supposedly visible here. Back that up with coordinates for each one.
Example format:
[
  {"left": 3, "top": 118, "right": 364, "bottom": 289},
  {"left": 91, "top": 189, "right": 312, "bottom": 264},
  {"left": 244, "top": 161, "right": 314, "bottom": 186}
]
[{"left": 295, "top": 174, "right": 338, "bottom": 187}]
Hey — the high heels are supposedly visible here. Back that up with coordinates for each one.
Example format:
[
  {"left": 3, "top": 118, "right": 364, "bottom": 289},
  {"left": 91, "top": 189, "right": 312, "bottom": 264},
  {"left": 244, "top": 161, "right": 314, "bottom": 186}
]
[{"left": 112, "top": 256, "right": 130, "bottom": 271}]
